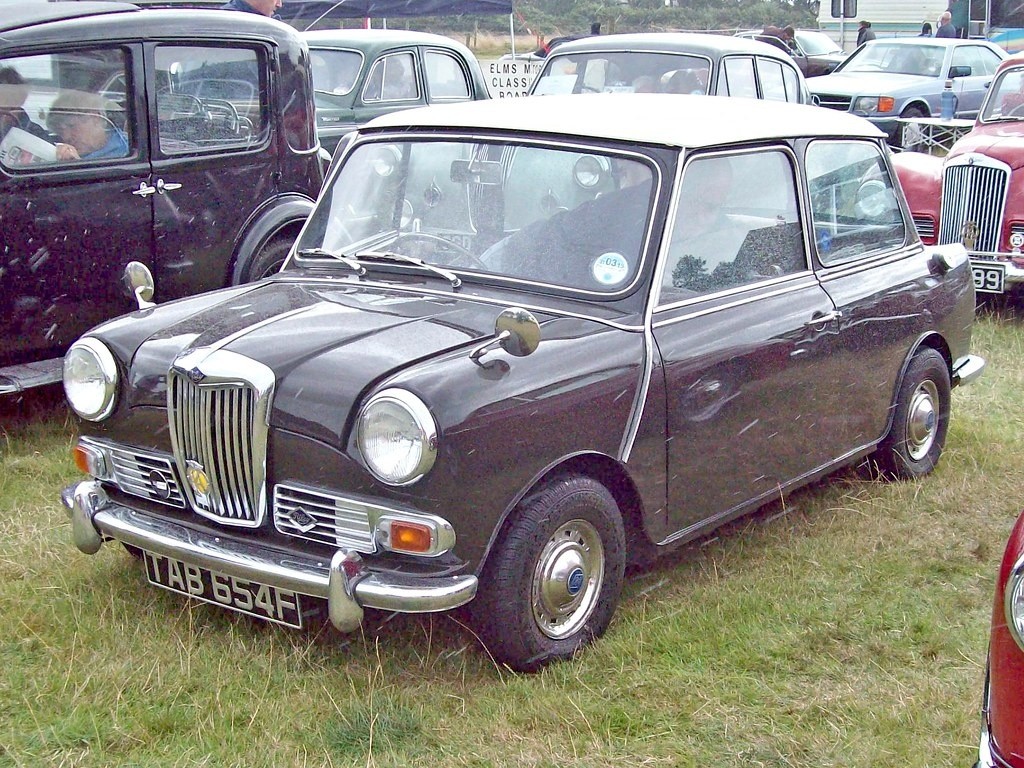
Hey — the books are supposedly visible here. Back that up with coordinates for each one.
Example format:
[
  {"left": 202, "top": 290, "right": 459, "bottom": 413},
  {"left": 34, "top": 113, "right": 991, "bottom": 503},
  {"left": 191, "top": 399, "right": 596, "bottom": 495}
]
[{"left": 0, "top": 127, "right": 57, "bottom": 166}]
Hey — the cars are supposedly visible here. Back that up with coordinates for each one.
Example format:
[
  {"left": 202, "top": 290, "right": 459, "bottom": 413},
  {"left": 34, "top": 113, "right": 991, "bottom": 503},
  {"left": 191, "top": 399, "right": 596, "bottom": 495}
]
[
  {"left": 296, "top": 27, "right": 494, "bottom": 166},
  {"left": 59, "top": 89, "right": 976, "bottom": 676},
  {"left": 0, "top": 0, "right": 330, "bottom": 399},
  {"left": 452, "top": 21, "right": 1024, "bottom": 303},
  {"left": 495, "top": 30, "right": 603, "bottom": 91}
]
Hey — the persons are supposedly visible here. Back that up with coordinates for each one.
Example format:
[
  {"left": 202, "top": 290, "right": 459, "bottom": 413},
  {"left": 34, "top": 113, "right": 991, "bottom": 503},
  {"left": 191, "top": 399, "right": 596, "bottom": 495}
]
[
  {"left": 0, "top": 65, "right": 56, "bottom": 147},
  {"left": 919, "top": 12, "right": 956, "bottom": 38},
  {"left": 503, "top": 154, "right": 743, "bottom": 290},
  {"left": 754, "top": 25, "right": 795, "bottom": 56},
  {"left": 46, "top": 90, "right": 129, "bottom": 161},
  {"left": 857, "top": 20, "right": 876, "bottom": 47},
  {"left": 632, "top": 69, "right": 706, "bottom": 94},
  {"left": 181, "top": 0, "right": 411, "bottom": 101}
]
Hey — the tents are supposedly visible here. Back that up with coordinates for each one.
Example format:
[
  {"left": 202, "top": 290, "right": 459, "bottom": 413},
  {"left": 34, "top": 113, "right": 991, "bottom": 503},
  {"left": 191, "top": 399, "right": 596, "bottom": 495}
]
[{"left": 271, "top": 0, "right": 516, "bottom": 60}]
